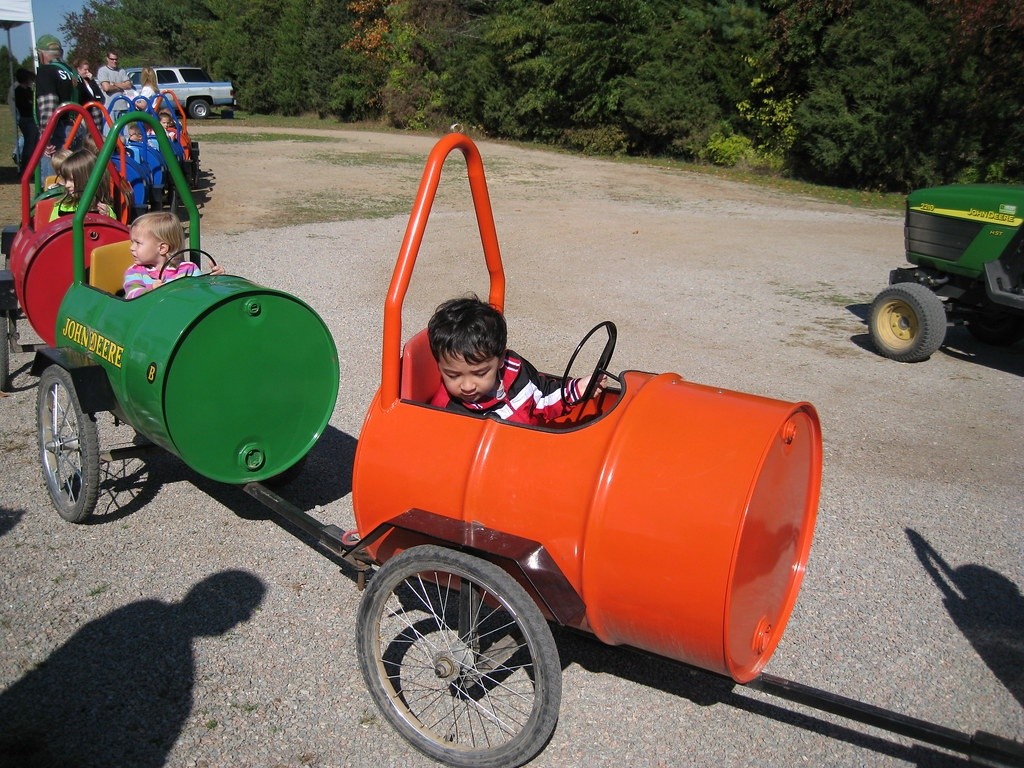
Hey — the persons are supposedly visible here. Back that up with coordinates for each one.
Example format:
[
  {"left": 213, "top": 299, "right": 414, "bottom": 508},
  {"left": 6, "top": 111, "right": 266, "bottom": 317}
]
[
  {"left": 13, "top": 32, "right": 224, "bottom": 299},
  {"left": 427, "top": 291, "right": 608, "bottom": 431}
]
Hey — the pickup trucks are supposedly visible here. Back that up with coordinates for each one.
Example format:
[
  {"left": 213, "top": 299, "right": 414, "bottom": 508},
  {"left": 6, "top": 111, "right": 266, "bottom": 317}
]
[{"left": 118, "top": 66, "right": 236, "bottom": 113}]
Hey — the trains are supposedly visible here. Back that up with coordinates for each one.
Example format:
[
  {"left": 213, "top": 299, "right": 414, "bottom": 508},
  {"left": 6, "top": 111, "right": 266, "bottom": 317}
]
[{"left": 1, "top": 86, "right": 1023, "bottom": 767}]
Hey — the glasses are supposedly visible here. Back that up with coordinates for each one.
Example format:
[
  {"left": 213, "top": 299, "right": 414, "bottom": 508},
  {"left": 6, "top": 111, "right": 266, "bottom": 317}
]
[
  {"left": 129, "top": 132, "right": 139, "bottom": 137},
  {"left": 108, "top": 58, "right": 118, "bottom": 61}
]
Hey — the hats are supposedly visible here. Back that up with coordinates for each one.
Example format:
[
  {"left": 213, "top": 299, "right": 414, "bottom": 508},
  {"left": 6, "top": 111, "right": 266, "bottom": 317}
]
[{"left": 34, "top": 34, "right": 62, "bottom": 50}]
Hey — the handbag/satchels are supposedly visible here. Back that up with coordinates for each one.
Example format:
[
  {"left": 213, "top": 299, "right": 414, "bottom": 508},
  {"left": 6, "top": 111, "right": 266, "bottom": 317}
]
[{"left": 124, "top": 88, "right": 140, "bottom": 108}]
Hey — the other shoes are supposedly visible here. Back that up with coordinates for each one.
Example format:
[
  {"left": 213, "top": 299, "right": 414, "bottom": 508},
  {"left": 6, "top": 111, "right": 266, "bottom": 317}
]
[{"left": 12, "top": 153, "right": 17, "bottom": 164}]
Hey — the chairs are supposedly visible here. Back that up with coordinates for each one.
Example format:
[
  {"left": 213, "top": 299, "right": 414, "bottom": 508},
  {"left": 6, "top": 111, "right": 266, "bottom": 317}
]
[
  {"left": 35, "top": 199, "right": 61, "bottom": 231},
  {"left": 44, "top": 175, "right": 61, "bottom": 191},
  {"left": 89, "top": 239, "right": 136, "bottom": 297},
  {"left": 401, "top": 328, "right": 450, "bottom": 409}
]
